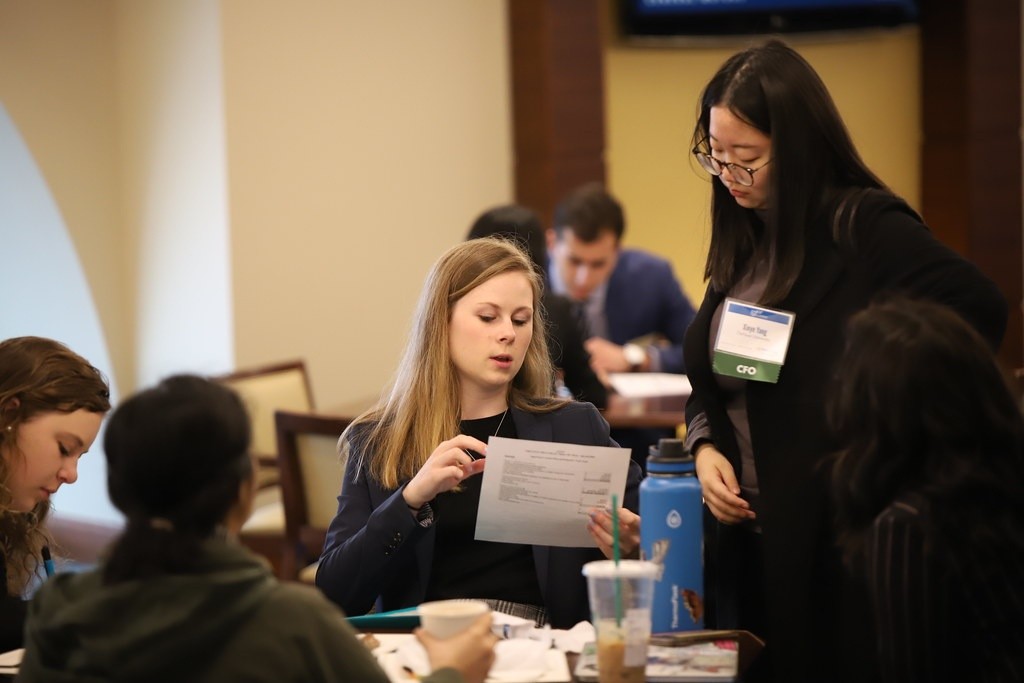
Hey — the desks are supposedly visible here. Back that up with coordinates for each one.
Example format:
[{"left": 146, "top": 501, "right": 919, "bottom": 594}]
[{"left": 557, "top": 370, "right": 693, "bottom": 426}]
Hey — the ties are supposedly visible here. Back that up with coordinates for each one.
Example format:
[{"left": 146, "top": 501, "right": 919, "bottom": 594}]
[{"left": 569, "top": 298, "right": 590, "bottom": 345}]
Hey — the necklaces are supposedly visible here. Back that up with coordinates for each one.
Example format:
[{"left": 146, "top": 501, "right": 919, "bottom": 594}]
[{"left": 456, "top": 407, "right": 510, "bottom": 461}]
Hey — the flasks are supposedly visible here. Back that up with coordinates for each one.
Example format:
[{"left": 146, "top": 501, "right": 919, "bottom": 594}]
[{"left": 638, "top": 439, "right": 705, "bottom": 633}]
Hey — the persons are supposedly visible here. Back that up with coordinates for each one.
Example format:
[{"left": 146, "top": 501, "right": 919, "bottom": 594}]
[
  {"left": 687, "top": 39, "right": 1024, "bottom": 683},
  {"left": 15, "top": 373, "right": 502, "bottom": 683},
  {"left": 0, "top": 335, "right": 111, "bottom": 654},
  {"left": 466, "top": 205, "right": 625, "bottom": 412},
  {"left": 547, "top": 181, "right": 698, "bottom": 372},
  {"left": 314, "top": 231, "right": 644, "bottom": 634}
]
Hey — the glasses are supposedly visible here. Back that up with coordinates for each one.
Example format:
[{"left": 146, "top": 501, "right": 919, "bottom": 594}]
[{"left": 692, "top": 134, "right": 776, "bottom": 186}]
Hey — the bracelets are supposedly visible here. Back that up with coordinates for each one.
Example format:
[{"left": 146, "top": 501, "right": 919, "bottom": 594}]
[{"left": 623, "top": 345, "right": 644, "bottom": 371}]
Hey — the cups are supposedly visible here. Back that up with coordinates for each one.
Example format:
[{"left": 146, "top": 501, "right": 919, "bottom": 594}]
[
  {"left": 582, "top": 560, "right": 659, "bottom": 683},
  {"left": 418, "top": 599, "right": 488, "bottom": 639}
]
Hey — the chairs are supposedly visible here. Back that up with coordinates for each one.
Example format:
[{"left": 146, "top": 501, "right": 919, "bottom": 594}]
[
  {"left": 275, "top": 410, "right": 366, "bottom": 580},
  {"left": 205, "top": 360, "right": 315, "bottom": 561}
]
